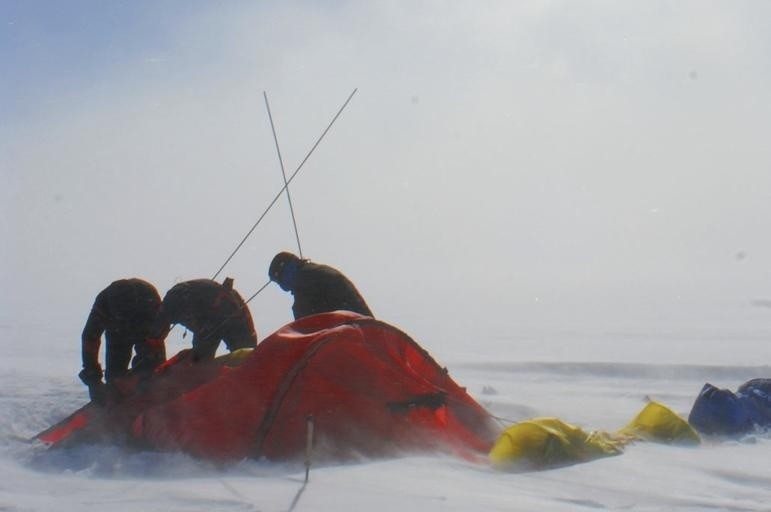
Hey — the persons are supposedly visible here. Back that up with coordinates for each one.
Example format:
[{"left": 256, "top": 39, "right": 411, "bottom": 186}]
[
  {"left": 77, "top": 277, "right": 165, "bottom": 401},
  {"left": 268, "top": 252, "right": 373, "bottom": 321},
  {"left": 142, "top": 280, "right": 256, "bottom": 368}
]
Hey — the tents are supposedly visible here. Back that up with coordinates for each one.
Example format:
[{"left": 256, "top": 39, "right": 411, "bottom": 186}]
[{"left": 24, "top": 309, "right": 505, "bottom": 465}]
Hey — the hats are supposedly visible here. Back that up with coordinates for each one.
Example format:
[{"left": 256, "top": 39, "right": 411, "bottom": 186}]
[
  {"left": 268, "top": 251, "right": 293, "bottom": 281},
  {"left": 108, "top": 279, "right": 137, "bottom": 317}
]
